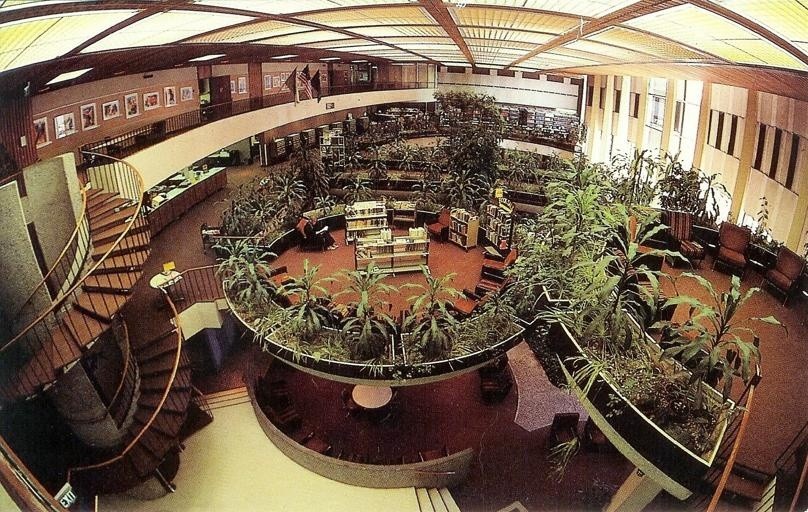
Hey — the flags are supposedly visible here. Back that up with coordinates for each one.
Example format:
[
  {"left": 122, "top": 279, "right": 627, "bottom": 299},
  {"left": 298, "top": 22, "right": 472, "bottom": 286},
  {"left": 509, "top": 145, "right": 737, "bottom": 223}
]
[
  {"left": 298, "top": 64, "right": 313, "bottom": 100},
  {"left": 285, "top": 68, "right": 300, "bottom": 107},
  {"left": 310, "top": 70, "right": 323, "bottom": 104}
]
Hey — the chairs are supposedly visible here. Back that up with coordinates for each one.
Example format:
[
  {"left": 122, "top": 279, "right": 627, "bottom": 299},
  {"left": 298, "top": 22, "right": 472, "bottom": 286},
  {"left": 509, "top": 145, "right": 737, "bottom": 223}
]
[
  {"left": 551, "top": 412, "right": 611, "bottom": 456},
  {"left": 294, "top": 217, "right": 327, "bottom": 253},
  {"left": 427, "top": 208, "right": 450, "bottom": 242},
  {"left": 479, "top": 352, "right": 514, "bottom": 403},
  {"left": 614, "top": 208, "right": 805, "bottom": 331},
  {"left": 256, "top": 376, "right": 451, "bottom": 466},
  {"left": 256, "top": 247, "right": 518, "bottom": 333}
]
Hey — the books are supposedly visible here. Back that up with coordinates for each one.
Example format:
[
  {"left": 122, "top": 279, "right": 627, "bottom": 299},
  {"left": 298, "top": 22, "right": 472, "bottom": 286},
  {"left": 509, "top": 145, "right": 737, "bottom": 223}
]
[
  {"left": 345, "top": 199, "right": 511, "bottom": 260},
  {"left": 316, "top": 226, "right": 328, "bottom": 235}
]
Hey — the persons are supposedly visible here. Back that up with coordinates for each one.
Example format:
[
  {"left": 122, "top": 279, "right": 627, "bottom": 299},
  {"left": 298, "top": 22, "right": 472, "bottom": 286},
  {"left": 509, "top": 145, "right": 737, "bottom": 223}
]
[{"left": 304, "top": 215, "right": 339, "bottom": 250}]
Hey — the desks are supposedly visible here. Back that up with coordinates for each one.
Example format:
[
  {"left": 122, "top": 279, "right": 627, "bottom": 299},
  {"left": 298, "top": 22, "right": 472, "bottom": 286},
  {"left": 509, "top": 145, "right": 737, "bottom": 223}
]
[{"left": 148, "top": 270, "right": 185, "bottom": 308}]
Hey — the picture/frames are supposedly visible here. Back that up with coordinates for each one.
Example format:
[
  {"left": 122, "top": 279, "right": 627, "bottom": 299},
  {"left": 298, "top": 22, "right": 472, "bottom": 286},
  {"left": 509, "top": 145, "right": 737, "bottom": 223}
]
[
  {"left": 32, "top": 85, "right": 193, "bottom": 150},
  {"left": 231, "top": 71, "right": 292, "bottom": 94}
]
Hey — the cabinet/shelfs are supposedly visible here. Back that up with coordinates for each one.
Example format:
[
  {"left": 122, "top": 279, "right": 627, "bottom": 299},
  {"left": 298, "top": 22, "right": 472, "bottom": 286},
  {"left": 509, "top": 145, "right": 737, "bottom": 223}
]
[
  {"left": 318, "top": 136, "right": 346, "bottom": 172},
  {"left": 447, "top": 196, "right": 515, "bottom": 253},
  {"left": 344, "top": 199, "right": 431, "bottom": 278}
]
